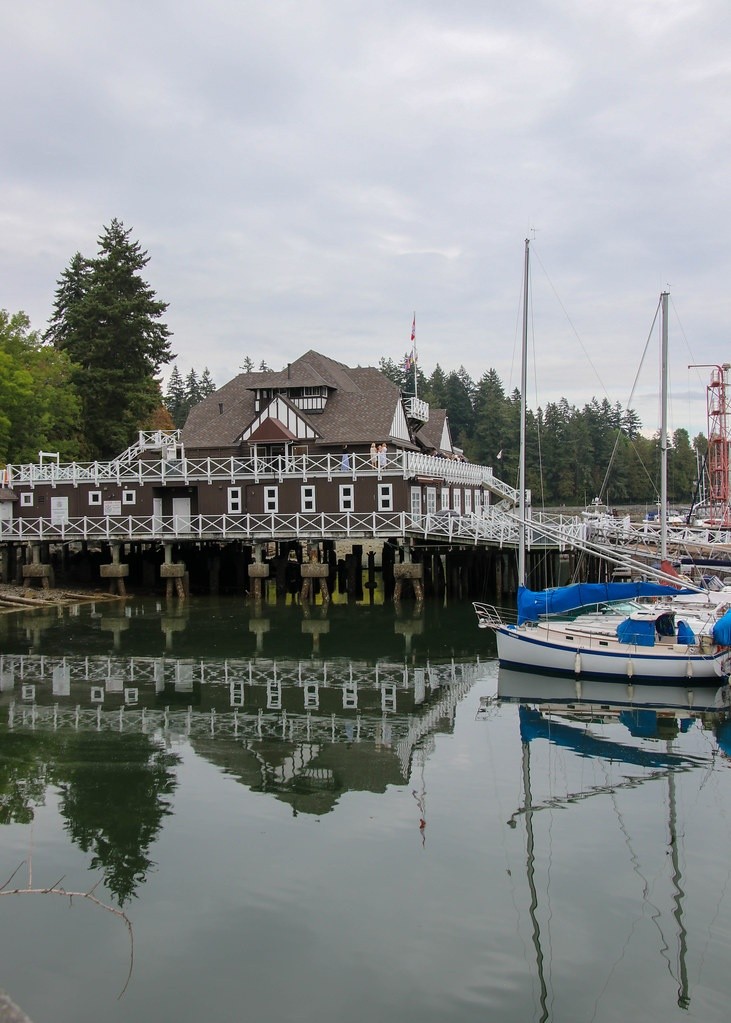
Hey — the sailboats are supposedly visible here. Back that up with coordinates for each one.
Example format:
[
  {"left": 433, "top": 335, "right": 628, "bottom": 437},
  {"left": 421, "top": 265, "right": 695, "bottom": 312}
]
[{"left": 472, "top": 233, "right": 731, "bottom": 681}]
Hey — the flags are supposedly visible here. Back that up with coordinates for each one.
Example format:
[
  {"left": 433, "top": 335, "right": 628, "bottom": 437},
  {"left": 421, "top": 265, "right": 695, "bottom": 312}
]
[
  {"left": 406, "top": 349, "right": 414, "bottom": 370},
  {"left": 411, "top": 318, "right": 416, "bottom": 341}
]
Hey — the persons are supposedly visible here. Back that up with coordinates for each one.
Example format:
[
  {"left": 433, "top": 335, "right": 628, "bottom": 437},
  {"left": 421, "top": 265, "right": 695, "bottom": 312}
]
[
  {"left": 341, "top": 443, "right": 349, "bottom": 471},
  {"left": 370, "top": 443, "right": 387, "bottom": 469}
]
